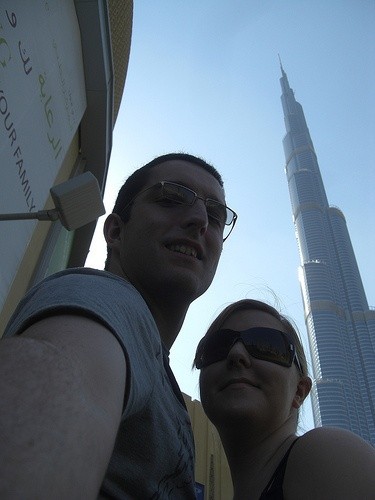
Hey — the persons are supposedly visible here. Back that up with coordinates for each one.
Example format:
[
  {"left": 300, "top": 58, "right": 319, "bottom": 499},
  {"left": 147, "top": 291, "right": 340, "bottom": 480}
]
[
  {"left": 191, "top": 298, "right": 375, "bottom": 500},
  {"left": 0, "top": 154, "right": 237, "bottom": 500}
]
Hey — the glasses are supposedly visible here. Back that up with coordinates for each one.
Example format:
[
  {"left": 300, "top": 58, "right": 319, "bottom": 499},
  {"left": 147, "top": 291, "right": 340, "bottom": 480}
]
[
  {"left": 194, "top": 326, "right": 303, "bottom": 377},
  {"left": 119, "top": 181, "right": 238, "bottom": 243}
]
